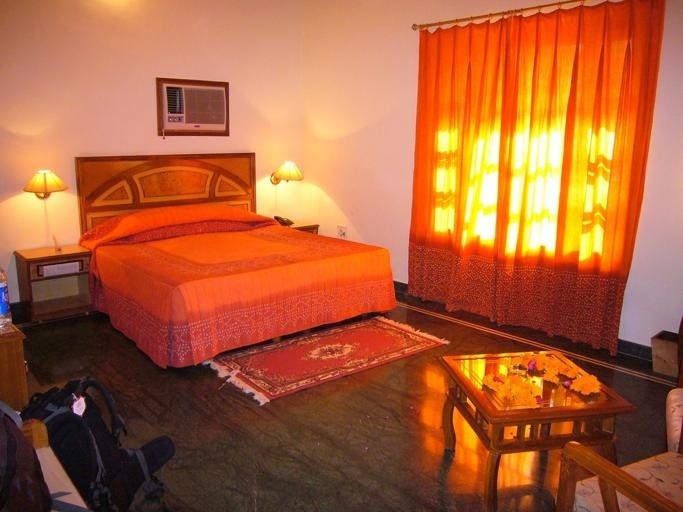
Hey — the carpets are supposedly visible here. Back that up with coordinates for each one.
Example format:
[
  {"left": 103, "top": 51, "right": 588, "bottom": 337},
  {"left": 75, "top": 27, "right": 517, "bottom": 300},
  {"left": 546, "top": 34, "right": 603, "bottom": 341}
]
[{"left": 203, "top": 314, "right": 451, "bottom": 405}]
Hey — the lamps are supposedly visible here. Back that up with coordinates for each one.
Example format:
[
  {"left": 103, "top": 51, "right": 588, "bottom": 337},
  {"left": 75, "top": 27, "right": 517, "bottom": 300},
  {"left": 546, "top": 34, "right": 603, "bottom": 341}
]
[
  {"left": 22, "top": 169, "right": 68, "bottom": 200},
  {"left": 268, "top": 161, "right": 304, "bottom": 186}
]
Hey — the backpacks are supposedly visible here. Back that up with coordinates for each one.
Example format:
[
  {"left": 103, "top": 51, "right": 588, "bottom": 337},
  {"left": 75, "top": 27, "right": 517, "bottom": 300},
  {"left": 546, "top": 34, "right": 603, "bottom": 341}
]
[{"left": 21, "top": 379, "right": 176, "bottom": 511}]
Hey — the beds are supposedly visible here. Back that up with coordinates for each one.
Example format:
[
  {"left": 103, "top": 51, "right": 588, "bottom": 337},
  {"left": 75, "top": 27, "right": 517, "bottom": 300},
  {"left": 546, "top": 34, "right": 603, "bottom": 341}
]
[{"left": 73, "top": 152, "right": 392, "bottom": 368}]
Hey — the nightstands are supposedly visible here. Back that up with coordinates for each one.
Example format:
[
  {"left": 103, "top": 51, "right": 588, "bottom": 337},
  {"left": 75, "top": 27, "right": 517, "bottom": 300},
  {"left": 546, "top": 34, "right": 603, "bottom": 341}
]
[{"left": 12, "top": 243, "right": 92, "bottom": 324}]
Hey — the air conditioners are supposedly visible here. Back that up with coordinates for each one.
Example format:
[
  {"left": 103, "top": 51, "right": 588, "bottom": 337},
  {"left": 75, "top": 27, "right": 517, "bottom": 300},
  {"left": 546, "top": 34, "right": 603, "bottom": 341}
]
[{"left": 162, "top": 84, "right": 227, "bottom": 131}]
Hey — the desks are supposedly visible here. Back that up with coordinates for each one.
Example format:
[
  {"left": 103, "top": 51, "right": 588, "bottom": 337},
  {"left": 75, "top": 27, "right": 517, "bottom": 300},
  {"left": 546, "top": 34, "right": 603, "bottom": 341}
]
[{"left": 437, "top": 347, "right": 638, "bottom": 512}]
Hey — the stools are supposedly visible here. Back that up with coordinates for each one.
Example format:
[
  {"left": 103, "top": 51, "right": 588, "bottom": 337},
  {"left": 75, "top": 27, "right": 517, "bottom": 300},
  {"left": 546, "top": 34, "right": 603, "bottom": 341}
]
[{"left": 553, "top": 441, "right": 681, "bottom": 512}]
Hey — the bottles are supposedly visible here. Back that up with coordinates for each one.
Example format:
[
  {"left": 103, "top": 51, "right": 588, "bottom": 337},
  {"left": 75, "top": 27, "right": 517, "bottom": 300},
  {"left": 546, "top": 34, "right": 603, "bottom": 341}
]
[{"left": 0, "top": 266, "right": 12, "bottom": 331}]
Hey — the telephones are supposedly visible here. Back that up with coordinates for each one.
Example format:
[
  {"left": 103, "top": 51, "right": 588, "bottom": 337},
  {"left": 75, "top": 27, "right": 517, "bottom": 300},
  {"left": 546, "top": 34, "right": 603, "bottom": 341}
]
[{"left": 275, "top": 216, "right": 293, "bottom": 226}]
